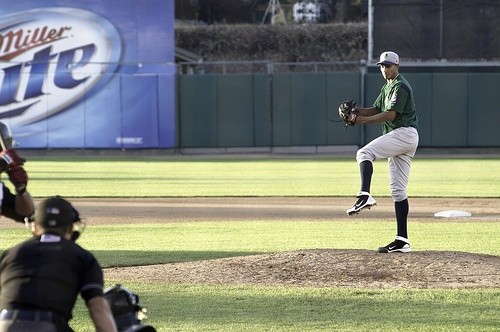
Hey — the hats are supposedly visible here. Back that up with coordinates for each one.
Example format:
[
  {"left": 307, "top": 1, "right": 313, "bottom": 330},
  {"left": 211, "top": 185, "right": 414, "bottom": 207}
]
[{"left": 376, "top": 51, "right": 399, "bottom": 65}]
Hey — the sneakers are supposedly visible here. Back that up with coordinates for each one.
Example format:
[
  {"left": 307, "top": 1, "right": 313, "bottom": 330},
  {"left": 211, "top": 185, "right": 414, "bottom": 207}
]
[
  {"left": 377, "top": 236, "right": 411, "bottom": 253},
  {"left": 346, "top": 192, "right": 377, "bottom": 216}
]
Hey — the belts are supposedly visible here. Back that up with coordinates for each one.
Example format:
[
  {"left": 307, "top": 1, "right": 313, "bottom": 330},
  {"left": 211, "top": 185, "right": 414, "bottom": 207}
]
[{"left": 0, "top": 311, "right": 60, "bottom": 320}]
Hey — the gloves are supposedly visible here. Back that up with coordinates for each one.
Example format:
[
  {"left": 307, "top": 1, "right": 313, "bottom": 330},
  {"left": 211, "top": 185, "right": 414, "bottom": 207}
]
[
  {"left": 0, "top": 150, "right": 26, "bottom": 172},
  {"left": 8, "top": 167, "right": 28, "bottom": 195}
]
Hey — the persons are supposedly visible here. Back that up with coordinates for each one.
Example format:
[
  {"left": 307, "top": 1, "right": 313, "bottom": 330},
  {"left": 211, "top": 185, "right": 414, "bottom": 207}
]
[
  {"left": 0, "top": 195, "right": 119, "bottom": 332},
  {"left": 103, "top": 285, "right": 157, "bottom": 331},
  {"left": 346, "top": 51, "right": 420, "bottom": 254},
  {"left": 0, "top": 119, "right": 37, "bottom": 223}
]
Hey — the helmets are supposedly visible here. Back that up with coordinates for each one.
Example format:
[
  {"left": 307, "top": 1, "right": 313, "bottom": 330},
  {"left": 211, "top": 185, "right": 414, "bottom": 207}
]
[
  {"left": 102, "top": 284, "right": 139, "bottom": 315},
  {"left": 34, "top": 196, "right": 80, "bottom": 227},
  {"left": 0, "top": 122, "right": 12, "bottom": 152}
]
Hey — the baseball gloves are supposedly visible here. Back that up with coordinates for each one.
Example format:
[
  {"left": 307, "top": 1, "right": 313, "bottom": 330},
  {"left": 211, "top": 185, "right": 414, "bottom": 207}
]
[{"left": 338, "top": 101, "right": 359, "bottom": 126}]
